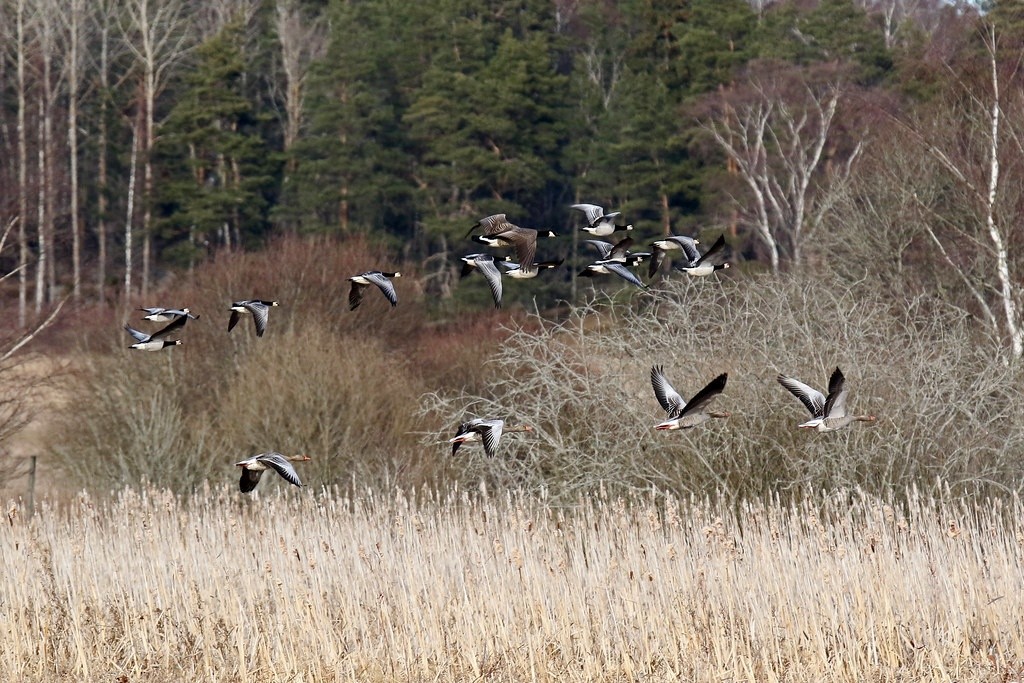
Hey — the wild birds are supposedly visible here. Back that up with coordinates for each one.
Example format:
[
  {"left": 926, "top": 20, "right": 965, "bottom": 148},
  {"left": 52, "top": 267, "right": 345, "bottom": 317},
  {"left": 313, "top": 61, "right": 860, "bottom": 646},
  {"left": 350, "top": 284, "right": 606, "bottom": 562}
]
[
  {"left": 346, "top": 270, "right": 403, "bottom": 310},
  {"left": 233, "top": 452, "right": 311, "bottom": 493},
  {"left": 124, "top": 306, "right": 200, "bottom": 351},
  {"left": 448, "top": 419, "right": 532, "bottom": 458},
  {"left": 461, "top": 203, "right": 730, "bottom": 306},
  {"left": 650, "top": 365, "right": 730, "bottom": 430},
  {"left": 778, "top": 367, "right": 876, "bottom": 434},
  {"left": 226, "top": 300, "right": 282, "bottom": 337}
]
